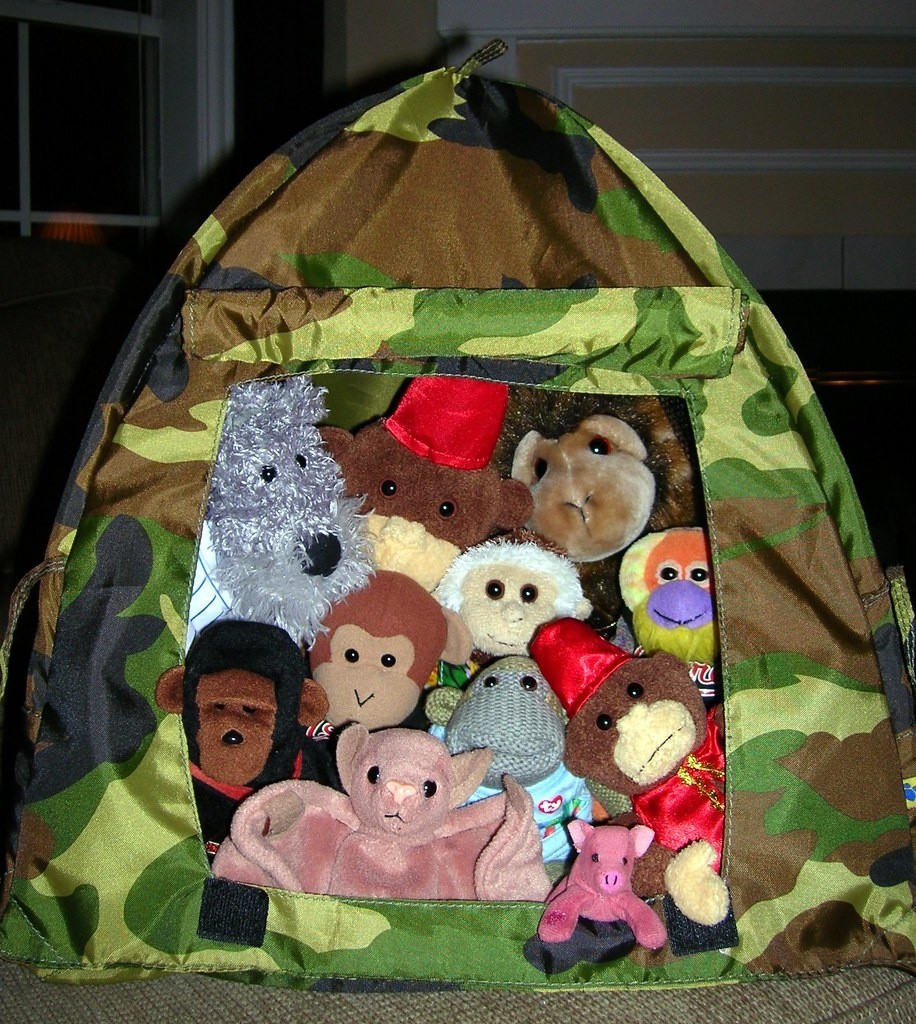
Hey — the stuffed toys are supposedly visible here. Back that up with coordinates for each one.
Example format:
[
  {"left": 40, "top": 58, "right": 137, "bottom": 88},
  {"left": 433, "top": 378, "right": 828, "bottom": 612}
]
[{"left": 156, "top": 374, "right": 735, "bottom": 950}]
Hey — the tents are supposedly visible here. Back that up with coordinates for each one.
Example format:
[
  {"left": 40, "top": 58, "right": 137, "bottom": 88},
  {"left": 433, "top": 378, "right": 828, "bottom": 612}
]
[{"left": 1, "top": 42, "right": 916, "bottom": 994}]
